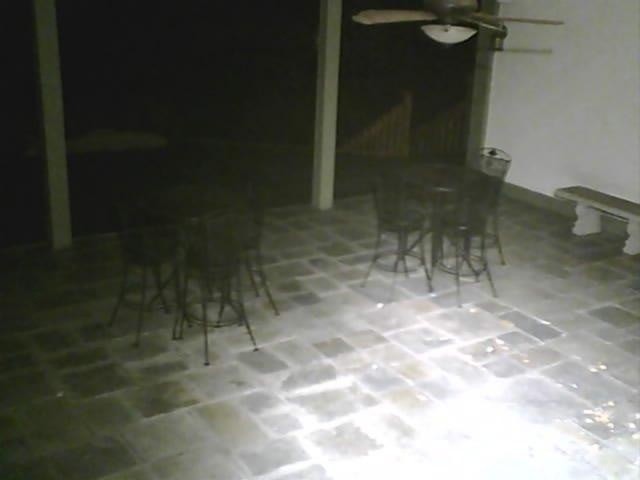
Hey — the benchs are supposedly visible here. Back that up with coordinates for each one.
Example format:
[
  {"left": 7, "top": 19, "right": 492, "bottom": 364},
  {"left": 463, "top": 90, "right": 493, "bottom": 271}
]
[{"left": 555, "top": 185, "right": 640, "bottom": 255}]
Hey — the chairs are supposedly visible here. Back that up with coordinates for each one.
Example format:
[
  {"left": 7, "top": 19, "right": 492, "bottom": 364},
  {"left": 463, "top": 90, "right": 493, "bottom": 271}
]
[
  {"left": 103, "top": 205, "right": 283, "bottom": 367},
  {"left": 363, "top": 147, "right": 510, "bottom": 309}
]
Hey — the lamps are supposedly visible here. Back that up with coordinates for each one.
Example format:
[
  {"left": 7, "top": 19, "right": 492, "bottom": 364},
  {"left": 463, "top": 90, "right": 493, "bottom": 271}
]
[{"left": 420, "top": 26, "right": 476, "bottom": 45}]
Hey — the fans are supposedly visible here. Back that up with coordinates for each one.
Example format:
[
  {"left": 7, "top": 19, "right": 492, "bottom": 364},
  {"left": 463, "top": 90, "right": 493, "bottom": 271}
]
[{"left": 352, "top": 0, "right": 565, "bottom": 33}]
